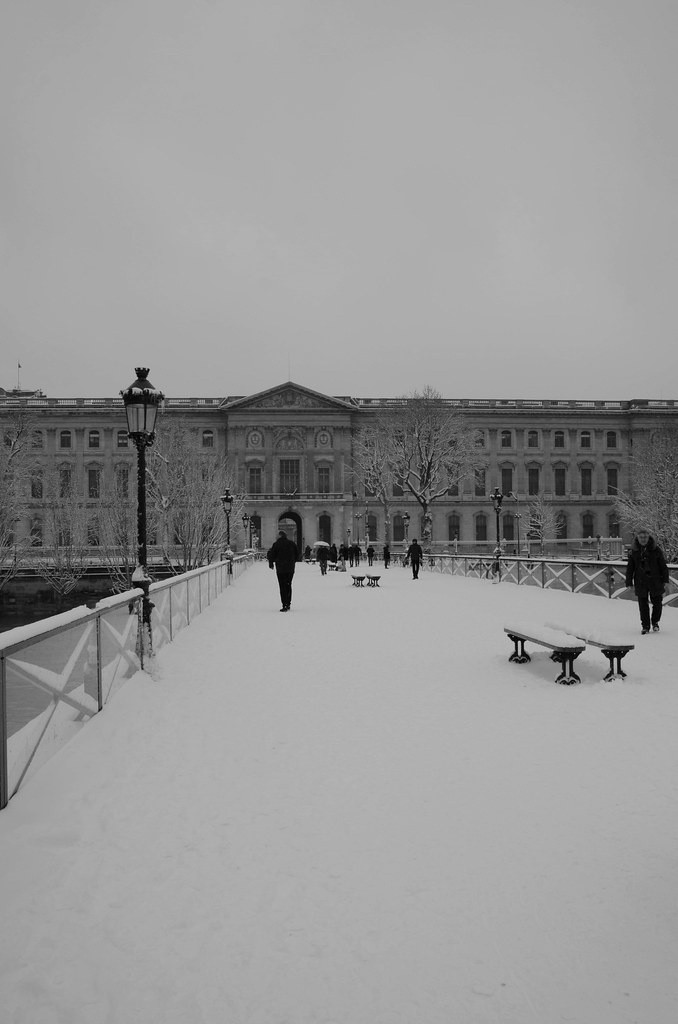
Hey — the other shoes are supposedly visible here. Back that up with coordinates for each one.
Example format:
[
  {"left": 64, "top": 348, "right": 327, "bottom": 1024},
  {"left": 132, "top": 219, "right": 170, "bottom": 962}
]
[
  {"left": 385, "top": 566, "right": 389, "bottom": 569},
  {"left": 653, "top": 624, "right": 659, "bottom": 631},
  {"left": 280, "top": 606, "right": 287, "bottom": 611},
  {"left": 287, "top": 606, "right": 290, "bottom": 609},
  {"left": 641, "top": 626, "right": 649, "bottom": 634},
  {"left": 413, "top": 576, "right": 418, "bottom": 580}
]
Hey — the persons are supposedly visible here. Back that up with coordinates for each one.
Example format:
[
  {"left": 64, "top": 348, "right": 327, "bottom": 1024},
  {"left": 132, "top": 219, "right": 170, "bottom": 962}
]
[
  {"left": 305, "top": 542, "right": 362, "bottom": 576},
  {"left": 406, "top": 539, "right": 422, "bottom": 579},
  {"left": 625, "top": 529, "right": 669, "bottom": 634},
  {"left": 367, "top": 545, "right": 374, "bottom": 566},
  {"left": 269, "top": 530, "right": 297, "bottom": 611},
  {"left": 384, "top": 545, "right": 390, "bottom": 569}
]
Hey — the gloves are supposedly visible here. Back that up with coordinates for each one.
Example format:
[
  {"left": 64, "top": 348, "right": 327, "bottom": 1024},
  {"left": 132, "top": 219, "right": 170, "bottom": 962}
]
[{"left": 269, "top": 564, "right": 274, "bottom": 569}]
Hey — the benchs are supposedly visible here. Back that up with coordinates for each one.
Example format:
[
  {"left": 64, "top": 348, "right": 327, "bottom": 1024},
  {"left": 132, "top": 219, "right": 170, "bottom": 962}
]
[
  {"left": 569, "top": 633, "right": 636, "bottom": 682},
  {"left": 366, "top": 575, "right": 381, "bottom": 587},
  {"left": 351, "top": 574, "right": 366, "bottom": 588},
  {"left": 503, "top": 623, "right": 587, "bottom": 685},
  {"left": 305, "top": 558, "right": 340, "bottom": 572}
]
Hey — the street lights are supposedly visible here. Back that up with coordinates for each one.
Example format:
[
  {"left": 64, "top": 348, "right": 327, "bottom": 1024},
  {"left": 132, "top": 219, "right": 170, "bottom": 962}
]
[
  {"left": 242, "top": 513, "right": 249, "bottom": 549},
  {"left": 346, "top": 528, "right": 352, "bottom": 547},
  {"left": 219, "top": 488, "right": 234, "bottom": 585},
  {"left": 119, "top": 367, "right": 165, "bottom": 674},
  {"left": 490, "top": 488, "right": 504, "bottom": 583},
  {"left": 402, "top": 511, "right": 410, "bottom": 551},
  {"left": 364, "top": 522, "right": 370, "bottom": 550}
]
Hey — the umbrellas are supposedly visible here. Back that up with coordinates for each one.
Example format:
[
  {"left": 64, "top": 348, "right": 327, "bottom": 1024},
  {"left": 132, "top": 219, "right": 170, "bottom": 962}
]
[{"left": 314, "top": 541, "right": 329, "bottom": 546}]
